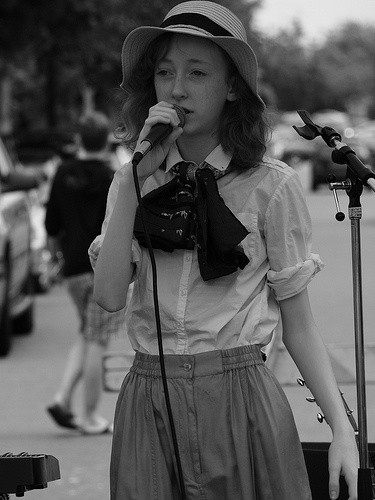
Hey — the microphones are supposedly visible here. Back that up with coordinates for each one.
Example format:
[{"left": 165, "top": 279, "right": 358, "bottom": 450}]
[{"left": 132, "top": 103, "right": 186, "bottom": 164}]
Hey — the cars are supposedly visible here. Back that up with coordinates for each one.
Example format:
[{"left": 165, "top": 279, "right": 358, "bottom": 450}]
[
  {"left": 274, "top": 111, "right": 352, "bottom": 164},
  {"left": 16, "top": 156, "right": 66, "bottom": 291},
  {"left": 309, "top": 136, "right": 369, "bottom": 193},
  {"left": 0, "top": 138, "right": 36, "bottom": 359},
  {"left": 76, "top": 130, "right": 135, "bottom": 168}
]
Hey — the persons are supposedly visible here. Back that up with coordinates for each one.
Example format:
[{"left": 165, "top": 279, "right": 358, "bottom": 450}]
[
  {"left": 45, "top": 109, "right": 121, "bottom": 436},
  {"left": 89, "top": 0, "right": 358, "bottom": 500}
]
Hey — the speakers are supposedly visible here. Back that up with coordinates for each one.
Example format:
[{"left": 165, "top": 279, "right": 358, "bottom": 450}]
[{"left": 300, "top": 441, "right": 375, "bottom": 500}]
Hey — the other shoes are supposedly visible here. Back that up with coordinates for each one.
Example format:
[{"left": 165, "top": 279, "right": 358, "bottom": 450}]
[
  {"left": 47, "top": 404, "right": 77, "bottom": 429},
  {"left": 72, "top": 416, "right": 110, "bottom": 434}
]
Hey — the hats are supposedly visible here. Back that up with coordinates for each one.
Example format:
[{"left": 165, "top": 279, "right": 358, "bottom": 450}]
[{"left": 119, "top": 0, "right": 266, "bottom": 115}]
